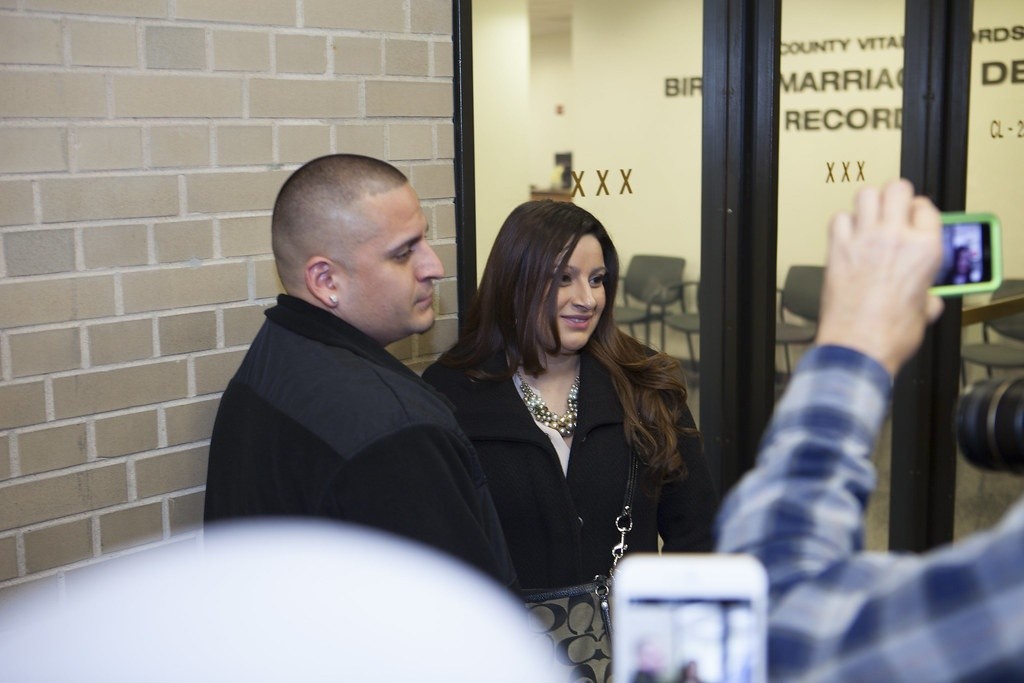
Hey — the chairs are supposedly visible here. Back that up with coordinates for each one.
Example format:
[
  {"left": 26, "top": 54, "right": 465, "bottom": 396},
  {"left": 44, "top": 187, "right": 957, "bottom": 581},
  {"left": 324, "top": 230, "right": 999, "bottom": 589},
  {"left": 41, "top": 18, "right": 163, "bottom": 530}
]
[
  {"left": 612, "top": 254, "right": 700, "bottom": 371},
  {"left": 960, "top": 278, "right": 1024, "bottom": 389},
  {"left": 774, "top": 265, "right": 826, "bottom": 380}
]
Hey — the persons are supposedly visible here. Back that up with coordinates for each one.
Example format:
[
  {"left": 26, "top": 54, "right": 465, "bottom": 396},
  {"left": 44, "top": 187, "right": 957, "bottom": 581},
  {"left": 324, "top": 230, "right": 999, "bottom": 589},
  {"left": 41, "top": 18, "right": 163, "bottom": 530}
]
[
  {"left": 202, "top": 150, "right": 521, "bottom": 593},
  {"left": 710, "top": 174, "right": 1024, "bottom": 683},
  {"left": 421, "top": 199, "right": 729, "bottom": 599}
]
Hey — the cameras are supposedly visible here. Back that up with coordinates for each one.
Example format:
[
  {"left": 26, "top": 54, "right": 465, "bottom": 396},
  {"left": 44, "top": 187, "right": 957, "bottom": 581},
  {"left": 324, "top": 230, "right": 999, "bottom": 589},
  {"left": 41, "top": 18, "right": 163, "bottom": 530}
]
[{"left": 957, "top": 377, "right": 1024, "bottom": 475}]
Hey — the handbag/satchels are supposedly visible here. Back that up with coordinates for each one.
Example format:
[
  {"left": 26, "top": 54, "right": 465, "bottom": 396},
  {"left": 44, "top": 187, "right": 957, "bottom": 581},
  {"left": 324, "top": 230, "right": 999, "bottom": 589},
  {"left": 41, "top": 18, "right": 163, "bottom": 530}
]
[{"left": 519, "top": 580, "right": 613, "bottom": 683}]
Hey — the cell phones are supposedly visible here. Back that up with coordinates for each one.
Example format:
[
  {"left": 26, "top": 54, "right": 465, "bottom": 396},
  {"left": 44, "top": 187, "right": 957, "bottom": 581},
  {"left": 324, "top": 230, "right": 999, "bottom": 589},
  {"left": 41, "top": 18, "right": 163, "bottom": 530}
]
[
  {"left": 612, "top": 552, "right": 769, "bottom": 683},
  {"left": 926, "top": 212, "right": 1001, "bottom": 296}
]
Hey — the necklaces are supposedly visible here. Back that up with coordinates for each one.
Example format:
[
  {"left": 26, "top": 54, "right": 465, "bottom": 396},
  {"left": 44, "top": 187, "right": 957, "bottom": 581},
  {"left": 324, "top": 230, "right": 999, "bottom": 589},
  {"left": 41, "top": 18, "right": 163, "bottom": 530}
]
[{"left": 513, "top": 368, "right": 581, "bottom": 438}]
